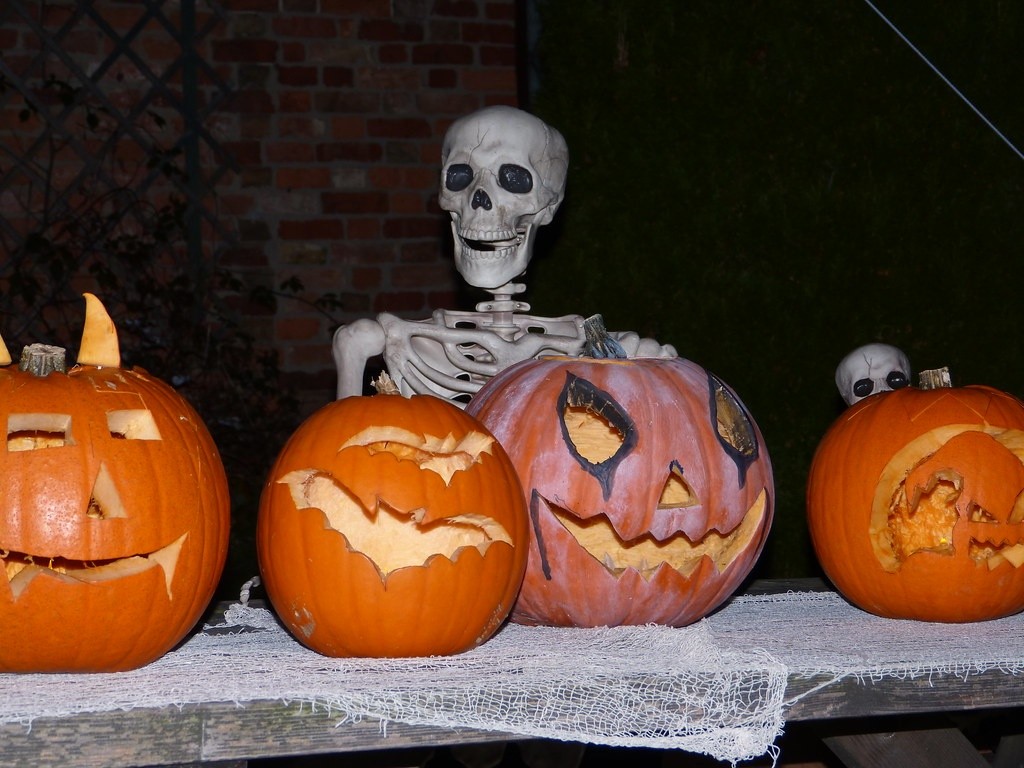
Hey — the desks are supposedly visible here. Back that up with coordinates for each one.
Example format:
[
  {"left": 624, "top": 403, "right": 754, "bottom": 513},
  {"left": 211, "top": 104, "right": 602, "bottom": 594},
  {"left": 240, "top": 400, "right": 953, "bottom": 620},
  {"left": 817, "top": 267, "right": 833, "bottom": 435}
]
[{"left": 0, "top": 578, "right": 1024, "bottom": 768}]
